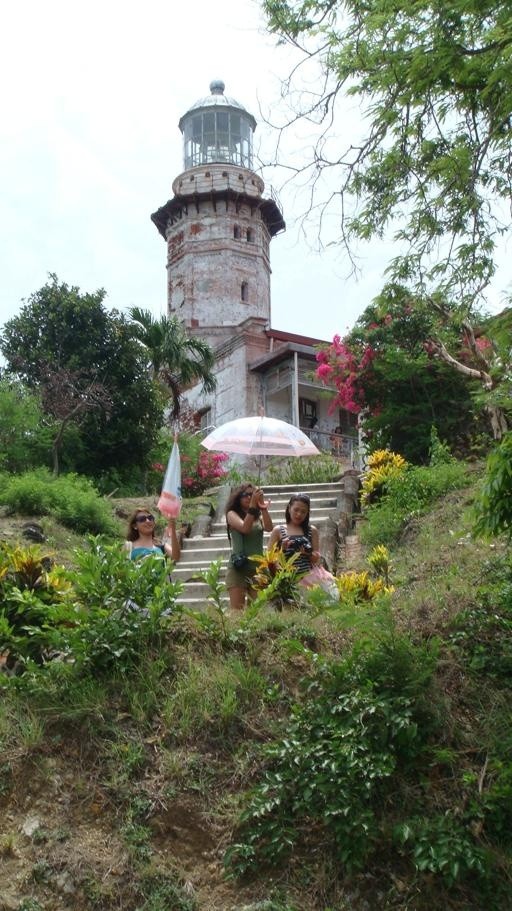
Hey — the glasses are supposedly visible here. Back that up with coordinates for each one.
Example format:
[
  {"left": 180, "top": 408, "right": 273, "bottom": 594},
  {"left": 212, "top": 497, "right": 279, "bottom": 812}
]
[
  {"left": 240, "top": 492, "right": 252, "bottom": 497},
  {"left": 135, "top": 515, "right": 154, "bottom": 522}
]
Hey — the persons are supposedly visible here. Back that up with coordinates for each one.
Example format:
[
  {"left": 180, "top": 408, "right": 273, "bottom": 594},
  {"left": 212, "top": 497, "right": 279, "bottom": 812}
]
[
  {"left": 122, "top": 507, "right": 183, "bottom": 584},
  {"left": 226, "top": 483, "right": 275, "bottom": 613},
  {"left": 267, "top": 493, "right": 321, "bottom": 613}
]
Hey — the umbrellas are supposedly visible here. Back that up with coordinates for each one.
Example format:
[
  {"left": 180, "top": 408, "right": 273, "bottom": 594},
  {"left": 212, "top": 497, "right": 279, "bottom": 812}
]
[
  {"left": 158, "top": 442, "right": 183, "bottom": 521},
  {"left": 201, "top": 417, "right": 322, "bottom": 490}
]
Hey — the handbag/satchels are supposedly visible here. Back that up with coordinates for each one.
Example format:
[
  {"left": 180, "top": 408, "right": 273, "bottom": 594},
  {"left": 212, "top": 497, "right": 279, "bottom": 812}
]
[
  {"left": 295, "top": 568, "right": 339, "bottom": 607},
  {"left": 231, "top": 552, "right": 249, "bottom": 569}
]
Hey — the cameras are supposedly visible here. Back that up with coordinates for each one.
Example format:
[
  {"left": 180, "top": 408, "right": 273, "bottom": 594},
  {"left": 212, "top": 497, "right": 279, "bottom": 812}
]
[
  {"left": 291, "top": 536, "right": 312, "bottom": 553},
  {"left": 233, "top": 556, "right": 249, "bottom": 568}
]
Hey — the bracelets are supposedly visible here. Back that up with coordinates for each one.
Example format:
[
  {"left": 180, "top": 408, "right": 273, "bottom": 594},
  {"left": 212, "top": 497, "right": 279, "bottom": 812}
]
[
  {"left": 306, "top": 553, "right": 312, "bottom": 559},
  {"left": 247, "top": 507, "right": 256, "bottom": 517}
]
